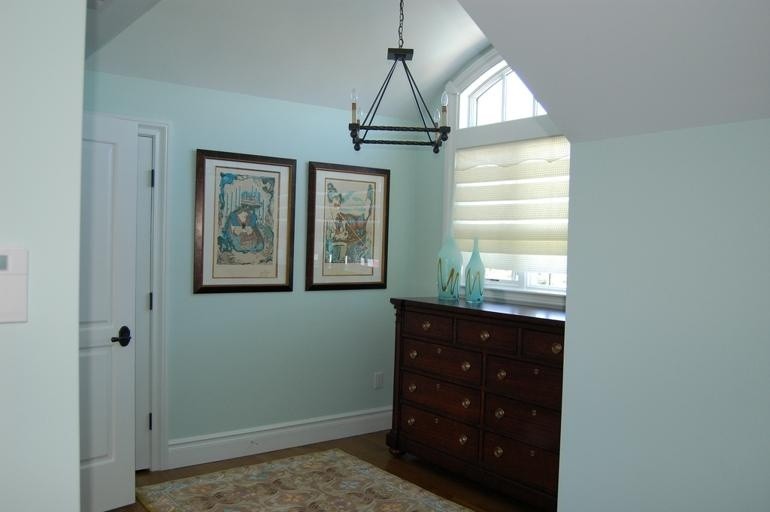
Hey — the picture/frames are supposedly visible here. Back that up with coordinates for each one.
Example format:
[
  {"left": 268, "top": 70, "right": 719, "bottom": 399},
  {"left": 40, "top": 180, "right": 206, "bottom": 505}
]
[
  {"left": 306, "top": 161, "right": 390, "bottom": 290},
  {"left": 194, "top": 149, "right": 296, "bottom": 294}
]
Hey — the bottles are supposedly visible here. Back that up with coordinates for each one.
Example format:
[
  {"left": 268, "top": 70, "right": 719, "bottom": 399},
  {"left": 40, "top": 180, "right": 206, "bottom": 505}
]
[
  {"left": 464, "top": 236, "right": 484, "bottom": 305},
  {"left": 437, "top": 231, "right": 462, "bottom": 299}
]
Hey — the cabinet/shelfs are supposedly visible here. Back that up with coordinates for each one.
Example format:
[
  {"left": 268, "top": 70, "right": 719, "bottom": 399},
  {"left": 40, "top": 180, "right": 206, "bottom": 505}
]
[{"left": 386, "top": 297, "right": 566, "bottom": 511}]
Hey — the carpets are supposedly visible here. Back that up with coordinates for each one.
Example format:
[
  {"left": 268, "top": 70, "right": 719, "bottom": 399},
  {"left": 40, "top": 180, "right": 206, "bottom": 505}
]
[{"left": 136, "top": 448, "right": 476, "bottom": 512}]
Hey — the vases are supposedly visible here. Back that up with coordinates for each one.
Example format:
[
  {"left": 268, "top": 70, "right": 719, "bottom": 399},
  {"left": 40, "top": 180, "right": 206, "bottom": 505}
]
[{"left": 435, "top": 234, "right": 485, "bottom": 304}]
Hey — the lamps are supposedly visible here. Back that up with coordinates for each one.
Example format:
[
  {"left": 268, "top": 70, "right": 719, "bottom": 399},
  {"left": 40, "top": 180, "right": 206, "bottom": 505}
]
[{"left": 349, "top": 0, "right": 451, "bottom": 153}]
